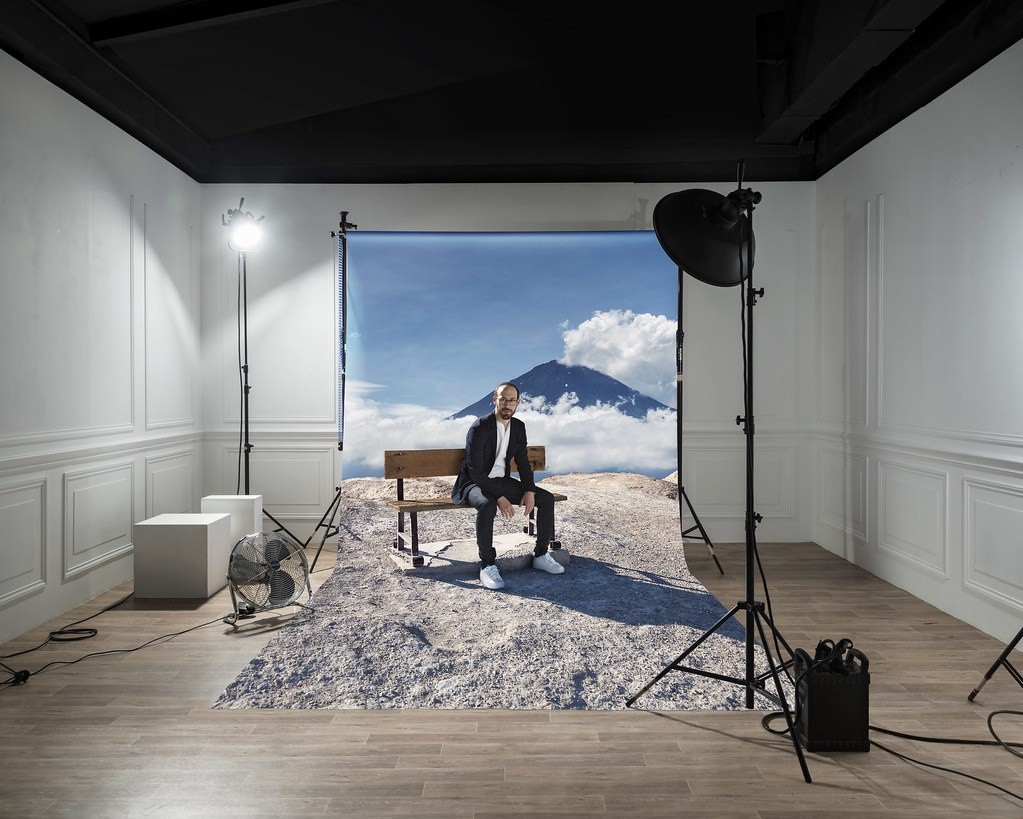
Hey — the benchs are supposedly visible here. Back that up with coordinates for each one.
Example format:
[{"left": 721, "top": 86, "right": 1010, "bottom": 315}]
[{"left": 384, "top": 445, "right": 569, "bottom": 565}]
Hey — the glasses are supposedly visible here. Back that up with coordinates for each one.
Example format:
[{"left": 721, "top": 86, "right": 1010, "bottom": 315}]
[{"left": 497, "top": 397, "right": 518, "bottom": 406}]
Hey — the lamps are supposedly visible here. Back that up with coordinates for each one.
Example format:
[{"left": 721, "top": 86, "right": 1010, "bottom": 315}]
[{"left": 220, "top": 196, "right": 306, "bottom": 549}]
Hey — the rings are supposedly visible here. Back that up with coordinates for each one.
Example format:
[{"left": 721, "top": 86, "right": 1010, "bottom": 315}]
[{"left": 531, "top": 508, "right": 533, "bottom": 510}]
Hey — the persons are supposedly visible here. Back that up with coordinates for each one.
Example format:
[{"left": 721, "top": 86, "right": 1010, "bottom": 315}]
[{"left": 450, "top": 381, "right": 565, "bottom": 589}]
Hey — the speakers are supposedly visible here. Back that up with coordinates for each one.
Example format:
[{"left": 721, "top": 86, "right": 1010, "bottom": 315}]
[{"left": 793, "top": 660, "right": 871, "bottom": 752}]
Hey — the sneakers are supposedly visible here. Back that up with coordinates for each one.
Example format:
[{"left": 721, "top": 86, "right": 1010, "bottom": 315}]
[
  {"left": 533, "top": 552, "right": 565, "bottom": 574},
  {"left": 479, "top": 564, "right": 505, "bottom": 589}
]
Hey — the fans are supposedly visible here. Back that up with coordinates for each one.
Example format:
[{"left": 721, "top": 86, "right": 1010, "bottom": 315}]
[{"left": 224, "top": 532, "right": 313, "bottom": 630}]
